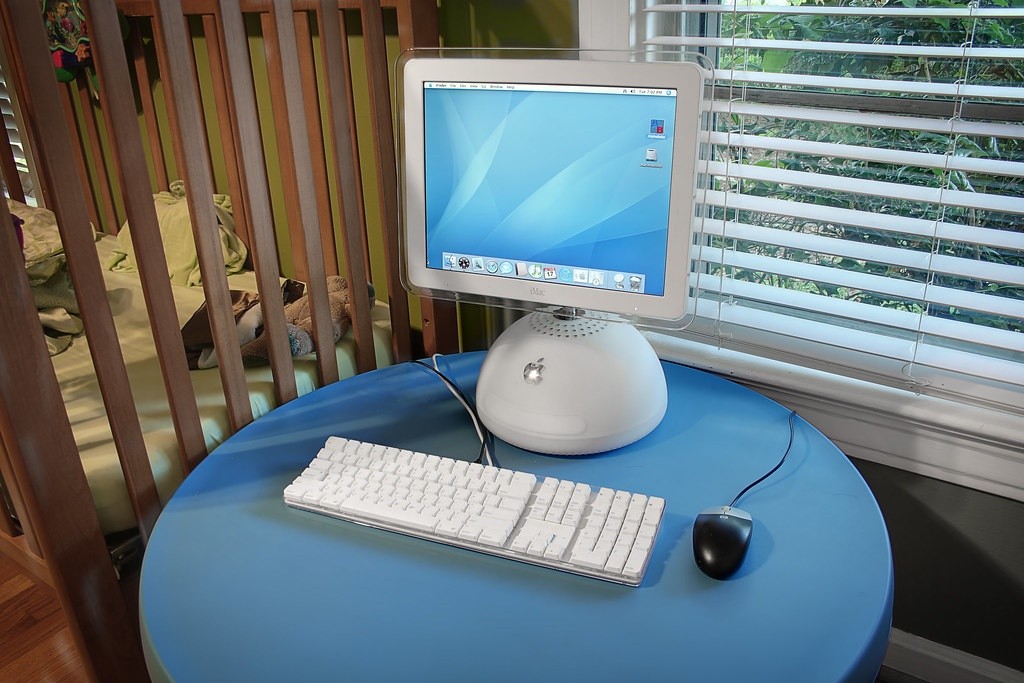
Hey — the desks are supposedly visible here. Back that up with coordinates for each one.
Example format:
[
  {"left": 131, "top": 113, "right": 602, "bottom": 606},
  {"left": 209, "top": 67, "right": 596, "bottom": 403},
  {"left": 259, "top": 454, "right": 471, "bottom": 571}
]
[{"left": 137, "top": 352, "right": 896, "bottom": 683}]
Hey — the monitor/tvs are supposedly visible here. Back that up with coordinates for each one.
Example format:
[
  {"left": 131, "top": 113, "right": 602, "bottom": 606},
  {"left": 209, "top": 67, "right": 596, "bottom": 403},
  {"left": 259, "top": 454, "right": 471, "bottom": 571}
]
[{"left": 395, "top": 47, "right": 714, "bottom": 457}]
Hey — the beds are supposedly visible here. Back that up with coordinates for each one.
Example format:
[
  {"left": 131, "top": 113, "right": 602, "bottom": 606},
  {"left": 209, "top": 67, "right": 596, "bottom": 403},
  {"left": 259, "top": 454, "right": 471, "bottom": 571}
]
[{"left": 0, "top": 0, "right": 486, "bottom": 683}]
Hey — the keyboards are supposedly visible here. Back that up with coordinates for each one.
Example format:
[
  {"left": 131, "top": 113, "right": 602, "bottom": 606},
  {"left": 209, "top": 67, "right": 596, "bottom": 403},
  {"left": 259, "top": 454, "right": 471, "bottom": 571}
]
[{"left": 285, "top": 437, "right": 666, "bottom": 587}]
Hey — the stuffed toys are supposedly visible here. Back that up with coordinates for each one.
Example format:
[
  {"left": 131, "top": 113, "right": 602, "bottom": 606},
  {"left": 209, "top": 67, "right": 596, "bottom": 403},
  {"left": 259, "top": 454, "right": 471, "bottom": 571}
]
[{"left": 242, "top": 274, "right": 376, "bottom": 366}]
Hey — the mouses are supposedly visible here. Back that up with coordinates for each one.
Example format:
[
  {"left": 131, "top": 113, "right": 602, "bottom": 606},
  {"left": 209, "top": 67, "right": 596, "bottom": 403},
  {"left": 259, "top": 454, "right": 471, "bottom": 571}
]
[{"left": 692, "top": 505, "right": 756, "bottom": 579}]
